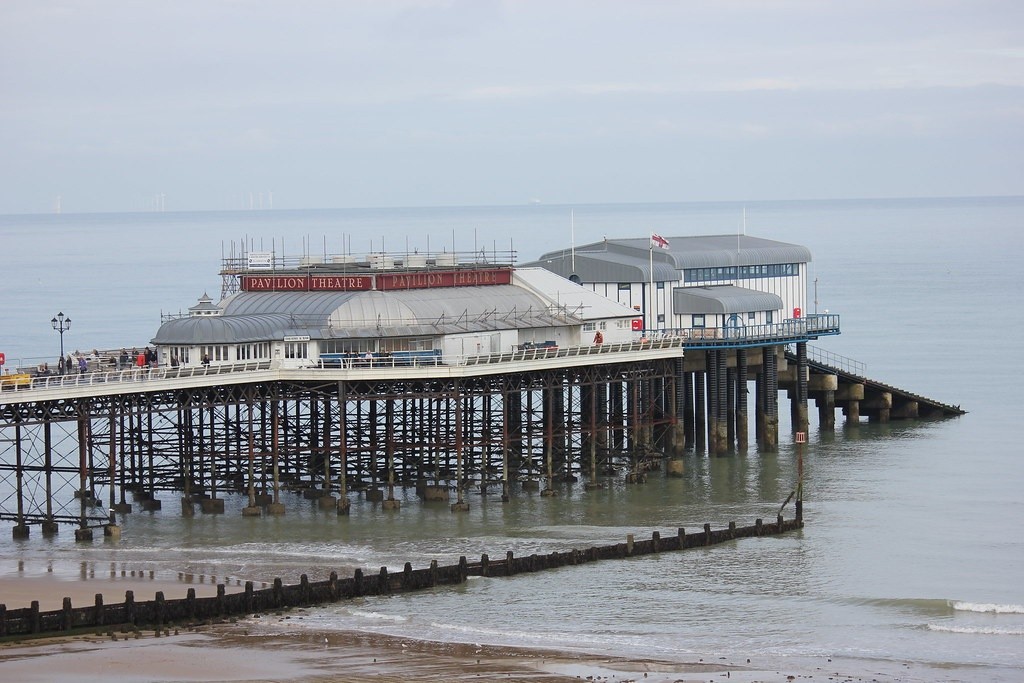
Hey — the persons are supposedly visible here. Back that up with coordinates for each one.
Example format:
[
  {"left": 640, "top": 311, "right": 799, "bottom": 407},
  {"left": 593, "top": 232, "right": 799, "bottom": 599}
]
[
  {"left": 353, "top": 351, "right": 360, "bottom": 367},
  {"left": 66, "top": 349, "right": 99, "bottom": 379},
  {"left": 593, "top": 331, "right": 603, "bottom": 351},
  {"left": 37, "top": 363, "right": 49, "bottom": 384},
  {"left": 379, "top": 350, "right": 393, "bottom": 367},
  {"left": 120, "top": 348, "right": 128, "bottom": 374},
  {"left": 171, "top": 356, "right": 179, "bottom": 378},
  {"left": 343, "top": 351, "right": 351, "bottom": 368},
  {"left": 364, "top": 351, "right": 373, "bottom": 367},
  {"left": 132, "top": 348, "right": 139, "bottom": 366},
  {"left": 144, "top": 347, "right": 157, "bottom": 373},
  {"left": 108, "top": 356, "right": 117, "bottom": 370},
  {"left": 203, "top": 355, "right": 210, "bottom": 375}
]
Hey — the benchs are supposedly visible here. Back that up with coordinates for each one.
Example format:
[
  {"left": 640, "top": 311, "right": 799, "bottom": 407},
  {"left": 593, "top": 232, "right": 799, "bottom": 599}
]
[
  {"left": 319, "top": 352, "right": 380, "bottom": 368},
  {"left": 380, "top": 348, "right": 442, "bottom": 366}
]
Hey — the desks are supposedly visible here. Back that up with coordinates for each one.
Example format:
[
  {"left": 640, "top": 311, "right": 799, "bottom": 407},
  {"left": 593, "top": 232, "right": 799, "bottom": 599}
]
[{"left": 16, "top": 367, "right": 47, "bottom": 378}]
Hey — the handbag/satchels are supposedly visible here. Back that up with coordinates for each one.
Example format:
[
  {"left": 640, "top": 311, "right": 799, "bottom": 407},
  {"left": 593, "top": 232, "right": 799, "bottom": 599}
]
[{"left": 84, "top": 366, "right": 87, "bottom": 371}]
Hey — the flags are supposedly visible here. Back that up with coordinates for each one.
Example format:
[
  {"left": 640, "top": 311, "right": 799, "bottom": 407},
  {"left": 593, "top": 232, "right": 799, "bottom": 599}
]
[{"left": 652, "top": 230, "right": 669, "bottom": 249}]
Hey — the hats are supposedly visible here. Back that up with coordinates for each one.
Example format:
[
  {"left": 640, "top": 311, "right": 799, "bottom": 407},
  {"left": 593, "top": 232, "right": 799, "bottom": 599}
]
[{"left": 145, "top": 347, "right": 149, "bottom": 349}]
